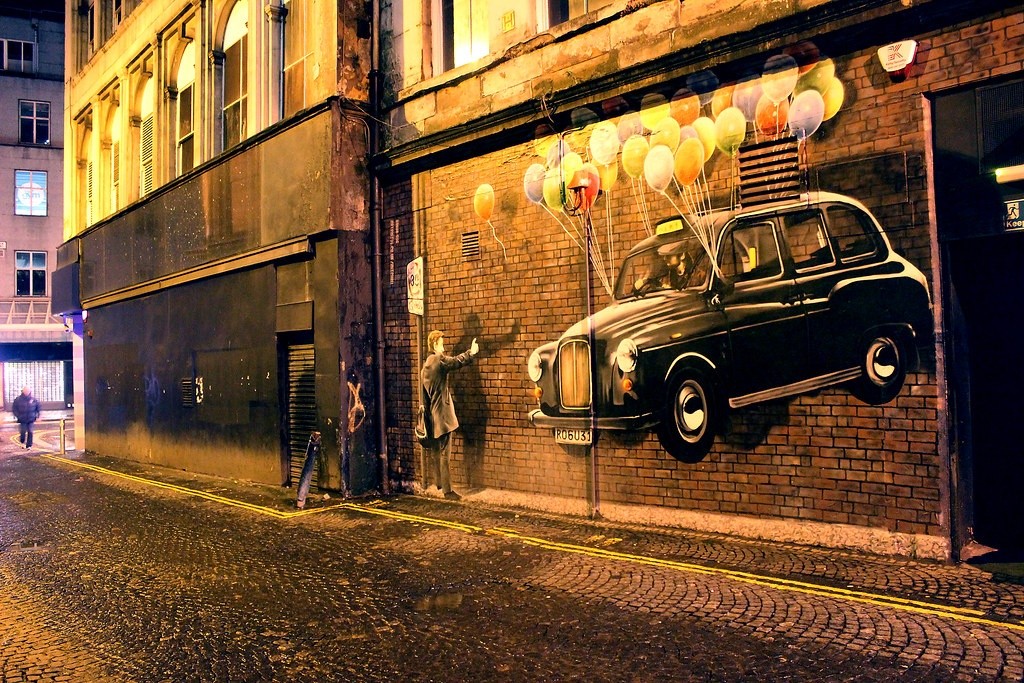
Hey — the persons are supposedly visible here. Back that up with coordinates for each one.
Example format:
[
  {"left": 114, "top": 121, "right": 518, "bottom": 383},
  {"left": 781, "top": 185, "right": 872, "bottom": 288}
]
[{"left": 13, "top": 386, "right": 41, "bottom": 450}]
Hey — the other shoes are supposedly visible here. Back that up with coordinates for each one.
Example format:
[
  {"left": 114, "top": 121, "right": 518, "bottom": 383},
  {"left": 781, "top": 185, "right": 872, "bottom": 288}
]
[
  {"left": 27, "top": 447, "right": 31, "bottom": 451},
  {"left": 21, "top": 445, "right": 24, "bottom": 449}
]
